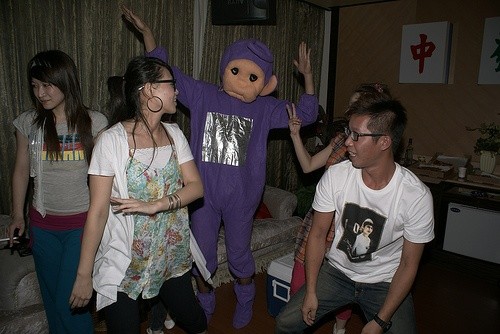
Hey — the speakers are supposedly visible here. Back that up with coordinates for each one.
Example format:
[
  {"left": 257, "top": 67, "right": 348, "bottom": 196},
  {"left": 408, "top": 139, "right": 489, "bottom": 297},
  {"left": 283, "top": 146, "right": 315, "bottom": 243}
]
[{"left": 210, "top": 0, "right": 276, "bottom": 26}]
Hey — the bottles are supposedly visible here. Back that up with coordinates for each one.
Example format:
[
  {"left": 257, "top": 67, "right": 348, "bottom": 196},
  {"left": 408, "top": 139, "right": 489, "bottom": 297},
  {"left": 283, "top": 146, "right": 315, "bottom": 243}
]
[{"left": 405, "top": 139, "right": 413, "bottom": 165}]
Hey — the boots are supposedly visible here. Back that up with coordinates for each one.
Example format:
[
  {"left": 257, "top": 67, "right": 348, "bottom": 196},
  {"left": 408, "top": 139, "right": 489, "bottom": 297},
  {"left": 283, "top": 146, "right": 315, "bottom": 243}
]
[
  {"left": 197, "top": 287, "right": 215, "bottom": 324},
  {"left": 232, "top": 277, "right": 256, "bottom": 329}
]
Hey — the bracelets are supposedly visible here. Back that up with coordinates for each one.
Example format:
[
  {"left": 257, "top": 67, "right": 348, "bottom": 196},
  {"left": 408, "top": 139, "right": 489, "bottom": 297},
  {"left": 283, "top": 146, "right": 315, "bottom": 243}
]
[{"left": 165, "top": 193, "right": 181, "bottom": 213}]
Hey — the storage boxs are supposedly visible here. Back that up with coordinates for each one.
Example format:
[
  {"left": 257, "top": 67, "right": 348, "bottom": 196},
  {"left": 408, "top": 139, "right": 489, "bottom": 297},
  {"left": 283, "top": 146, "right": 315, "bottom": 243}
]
[{"left": 267, "top": 250, "right": 295, "bottom": 317}]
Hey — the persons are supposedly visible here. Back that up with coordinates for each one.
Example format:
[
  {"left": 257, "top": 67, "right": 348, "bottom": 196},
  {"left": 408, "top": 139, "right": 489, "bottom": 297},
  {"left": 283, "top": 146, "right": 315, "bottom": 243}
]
[
  {"left": 275, "top": 92, "right": 435, "bottom": 334},
  {"left": 9, "top": 49, "right": 109, "bottom": 334},
  {"left": 122, "top": 4, "right": 318, "bottom": 329},
  {"left": 69, "top": 56, "right": 207, "bottom": 334},
  {"left": 286, "top": 84, "right": 391, "bottom": 334}
]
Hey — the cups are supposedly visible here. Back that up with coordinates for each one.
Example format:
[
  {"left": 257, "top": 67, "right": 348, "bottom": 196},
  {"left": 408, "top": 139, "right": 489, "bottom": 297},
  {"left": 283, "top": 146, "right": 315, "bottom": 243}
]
[{"left": 458, "top": 168, "right": 467, "bottom": 178}]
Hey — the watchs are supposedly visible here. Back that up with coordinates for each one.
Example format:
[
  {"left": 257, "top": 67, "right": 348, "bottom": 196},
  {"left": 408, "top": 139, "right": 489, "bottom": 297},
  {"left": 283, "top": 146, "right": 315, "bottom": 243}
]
[{"left": 374, "top": 315, "right": 392, "bottom": 332}]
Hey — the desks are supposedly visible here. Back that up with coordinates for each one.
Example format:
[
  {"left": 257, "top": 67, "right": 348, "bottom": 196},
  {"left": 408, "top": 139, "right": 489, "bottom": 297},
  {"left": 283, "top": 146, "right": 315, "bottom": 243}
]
[{"left": 404, "top": 157, "right": 500, "bottom": 212}]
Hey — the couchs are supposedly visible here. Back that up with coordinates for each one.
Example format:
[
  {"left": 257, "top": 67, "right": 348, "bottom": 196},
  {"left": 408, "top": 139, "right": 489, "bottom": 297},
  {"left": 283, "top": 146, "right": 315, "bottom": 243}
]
[{"left": 0, "top": 184, "right": 304, "bottom": 334}]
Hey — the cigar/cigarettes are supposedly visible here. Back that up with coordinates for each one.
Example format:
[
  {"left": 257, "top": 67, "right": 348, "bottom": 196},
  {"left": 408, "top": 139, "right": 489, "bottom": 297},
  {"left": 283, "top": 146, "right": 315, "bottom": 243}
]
[{"left": 0, "top": 237, "right": 17, "bottom": 241}]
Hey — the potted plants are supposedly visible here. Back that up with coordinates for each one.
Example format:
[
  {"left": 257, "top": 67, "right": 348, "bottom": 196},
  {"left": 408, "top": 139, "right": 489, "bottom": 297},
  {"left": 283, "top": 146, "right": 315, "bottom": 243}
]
[{"left": 466, "top": 112, "right": 500, "bottom": 174}]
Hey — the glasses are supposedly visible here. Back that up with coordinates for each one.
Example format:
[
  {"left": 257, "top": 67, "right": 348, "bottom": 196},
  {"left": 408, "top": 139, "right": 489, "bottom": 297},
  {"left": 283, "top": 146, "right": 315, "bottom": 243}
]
[
  {"left": 344, "top": 126, "right": 387, "bottom": 141},
  {"left": 138, "top": 79, "right": 176, "bottom": 92}
]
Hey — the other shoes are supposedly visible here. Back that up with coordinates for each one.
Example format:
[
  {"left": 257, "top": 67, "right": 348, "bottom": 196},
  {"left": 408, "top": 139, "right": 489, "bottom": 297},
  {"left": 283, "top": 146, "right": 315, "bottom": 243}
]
[
  {"left": 333, "top": 323, "right": 345, "bottom": 334},
  {"left": 147, "top": 327, "right": 164, "bottom": 334},
  {"left": 164, "top": 319, "right": 175, "bottom": 329}
]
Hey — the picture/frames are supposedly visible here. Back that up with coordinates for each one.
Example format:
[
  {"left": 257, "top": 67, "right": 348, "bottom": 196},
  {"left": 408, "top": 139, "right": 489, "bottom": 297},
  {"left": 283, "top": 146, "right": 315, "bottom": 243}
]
[
  {"left": 399, "top": 21, "right": 453, "bottom": 85},
  {"left": 478, "top": 17, "right": 500, "bottom": 84}
]
